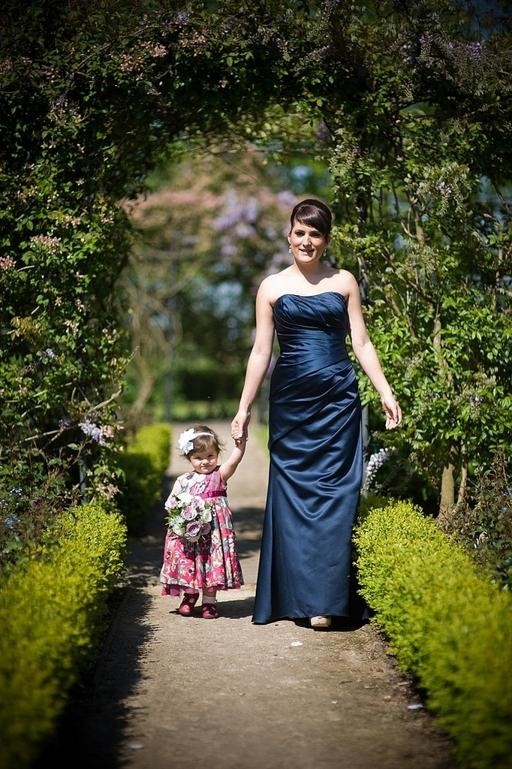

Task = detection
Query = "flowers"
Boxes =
[165,493,214,545]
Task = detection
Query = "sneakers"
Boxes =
[178,593,218,619]
[311,615,331,627]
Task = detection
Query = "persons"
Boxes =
[227,199,405,627]
[154,424,245,619]
